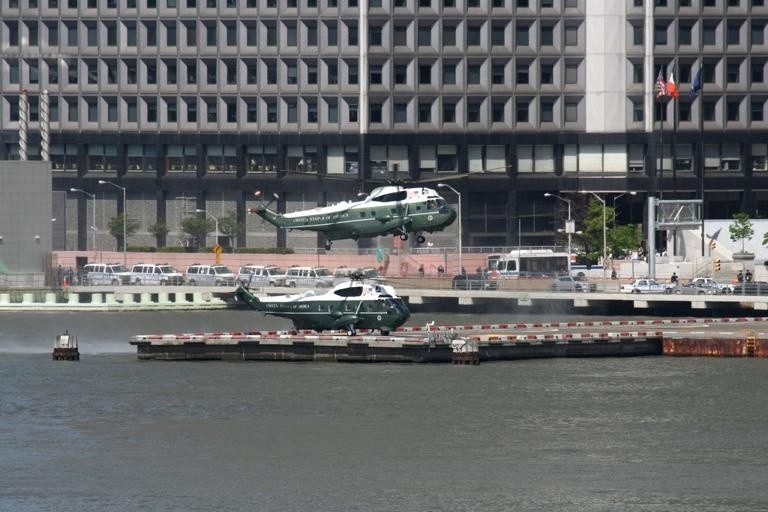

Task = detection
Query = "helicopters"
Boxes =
[244,164,515,250]
[209,266,413,336]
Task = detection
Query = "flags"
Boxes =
[687,55,703,100]
[665,54,681,99]
[654,63,666,100]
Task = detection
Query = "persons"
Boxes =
[476,265,481,274]
[55,264,63,286]
[438,264,444,274]
[75,267,85,284]
[65,266,75,284]
[744,269,752,283]
[671,272,678,283]
[492,267,497,277]
[737,269,743,283]
[418,264,425,277]
[483,267,489,276]
[461,266,466,274]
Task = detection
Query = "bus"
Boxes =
[485,247,574,281]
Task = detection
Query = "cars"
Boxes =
[617,277,675,294]
[549,275,597,294]
[690,277,738,295]
[731,281,768,296]
[670,282,722,297]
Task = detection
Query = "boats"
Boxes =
[50,328,81,362]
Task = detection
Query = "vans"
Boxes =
[331,267,388,288]
[86,263,131,286]
[184,264,238,286]
[284,267,335,288]
[451,273,498,291]
[129,263,185,286]
[238,267,285,286]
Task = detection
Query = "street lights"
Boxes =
[194,208,219,246]
[543,193,573,276]
[69,187,97,251]
[437,183,462,275]
[97,180,128,263]
[581,189,638,271]
